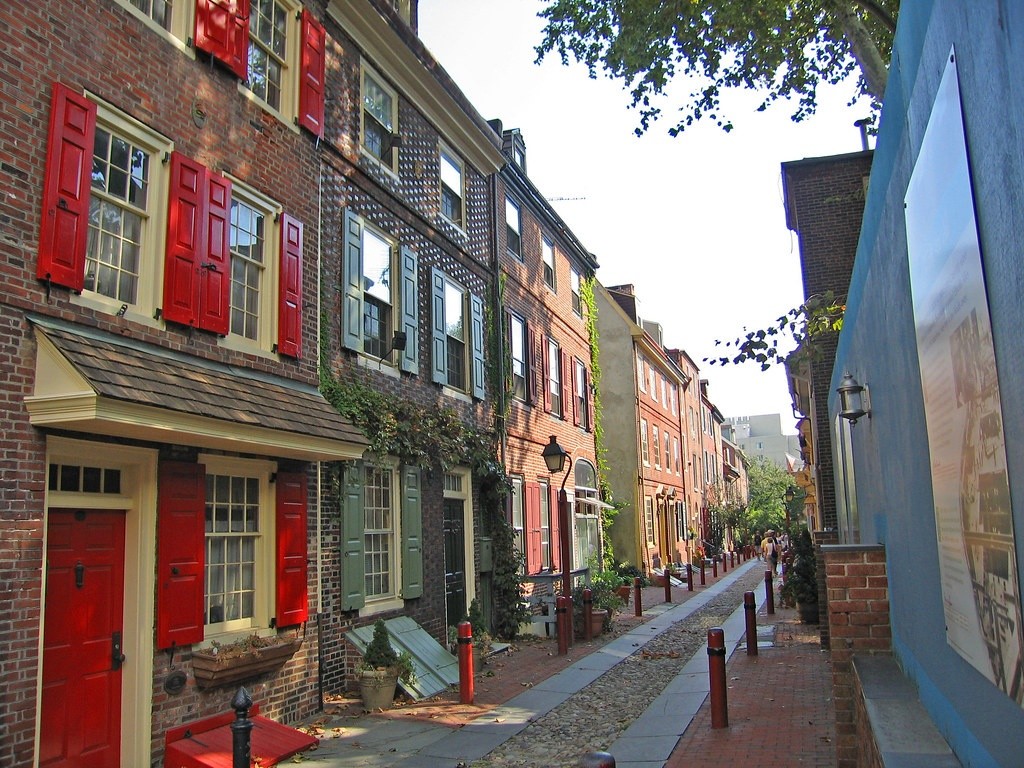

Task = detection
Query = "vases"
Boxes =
[656,576,665,587]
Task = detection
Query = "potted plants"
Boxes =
[789,529,819,625]
[613,576,631,607]
[574,570,606,636]
[458,596,487,671]
[669,564,681,579]
[599,570,622,630]
[353,617,417,712]
[192,630,305,691]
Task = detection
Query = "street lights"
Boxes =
[540,433,574,645]
[782,486,795,549]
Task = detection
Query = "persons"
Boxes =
[753,529,788,576]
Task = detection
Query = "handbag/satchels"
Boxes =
[771,544,778,559]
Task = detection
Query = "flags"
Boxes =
[785,454,795,471]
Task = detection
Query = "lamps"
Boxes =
[801,451,810,465]
[836,369,872,428]
[378,331,406,371]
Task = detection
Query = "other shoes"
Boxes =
[772,573,776,578]
